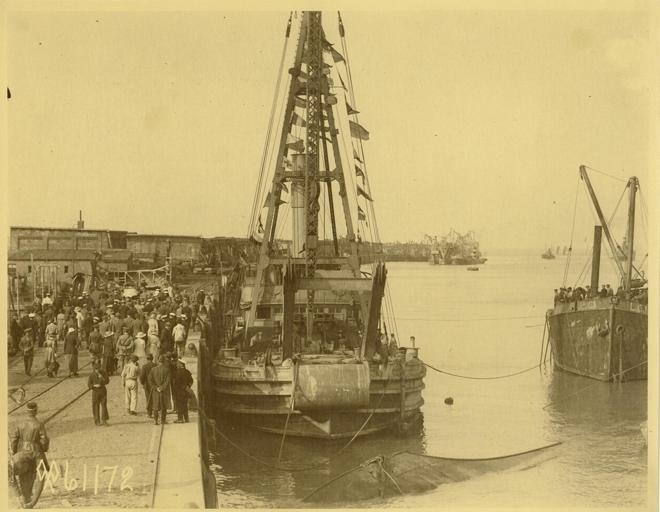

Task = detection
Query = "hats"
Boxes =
[93,317,99,321]
[123,327,129,332]
[69,328,75,333]
[24,328,32,332]
[46,293,51,296]
[92,360,101,369]
[151,313,187,320]
[104,332,113,337]
[106,305,114,309]
[74,307,81,312]
[26,402,39,410]
[135,332,146,338]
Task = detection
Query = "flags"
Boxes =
[284,132,305,153]
[249,232,263,247]
[330,45,346,65]
[349,120,370,141]
[291,111,306,127]
[281,155,294,171]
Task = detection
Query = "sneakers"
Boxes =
[97,420,108,426]
[128,410,189,425]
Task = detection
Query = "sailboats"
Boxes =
[191,8,425,446]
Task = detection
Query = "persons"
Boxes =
[10,278,206,430]
[552,283,614,307]
[9,401,50,510]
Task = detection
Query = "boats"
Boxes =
[429,230,637,264]
[541,162,648,387]
